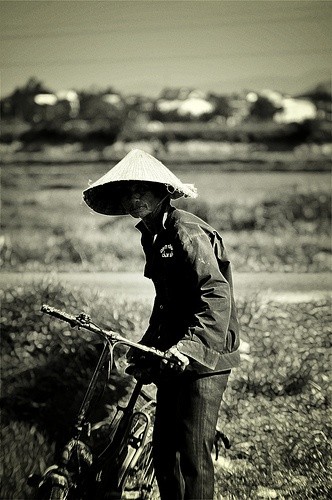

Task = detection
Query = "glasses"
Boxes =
[119,184,153,202]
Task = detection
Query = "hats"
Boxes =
[83,149,198,215]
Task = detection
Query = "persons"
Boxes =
[82,148,241,500]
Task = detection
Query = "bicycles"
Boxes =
[28,305,231,500]
